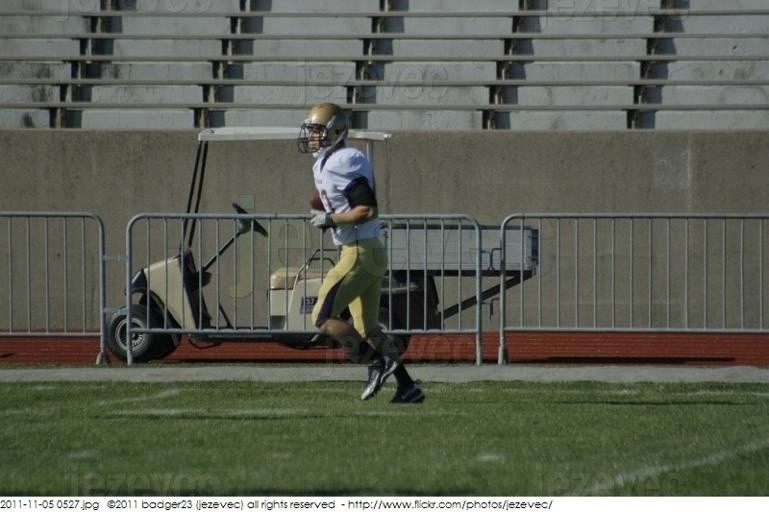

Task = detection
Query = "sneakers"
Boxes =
[360,351,426,405]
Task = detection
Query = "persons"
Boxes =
[295,101,427,404]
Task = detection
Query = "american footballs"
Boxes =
[309,191,325,210]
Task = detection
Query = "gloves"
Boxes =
[309,209,332,229]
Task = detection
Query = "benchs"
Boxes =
[2,1,767,133]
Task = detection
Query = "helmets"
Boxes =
[297,102,349,155]
